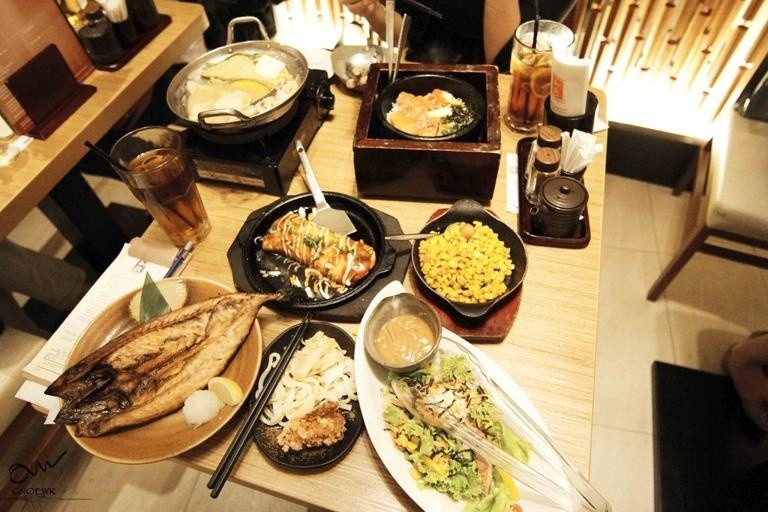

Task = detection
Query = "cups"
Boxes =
[364,296,442,373]
[560,149,589,180]
[525,174,586,238]
[544,89,600,140]
[107,129,209,253]
[502,19,576,133]
[74,0,159,61]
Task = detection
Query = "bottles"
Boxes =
[523,126,561,207]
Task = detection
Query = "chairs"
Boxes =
[647,52,768,303]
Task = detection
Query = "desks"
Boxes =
[1,0,211,339]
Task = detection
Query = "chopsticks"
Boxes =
[201,310,316,500]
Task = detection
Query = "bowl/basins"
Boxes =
[333,43,398,93]
[408,200,528,321]
[374,73,487,142]
[244,189,399,310]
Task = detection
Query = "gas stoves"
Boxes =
[131,62,340,195]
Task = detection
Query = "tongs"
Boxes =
[386,333,614,512]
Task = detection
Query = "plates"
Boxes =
[353,278,597,512]
[248,321,366,468]
[62,276,261,465]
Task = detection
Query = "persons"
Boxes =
[341,0,561,74]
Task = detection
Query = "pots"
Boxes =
[162,12,313,149]
